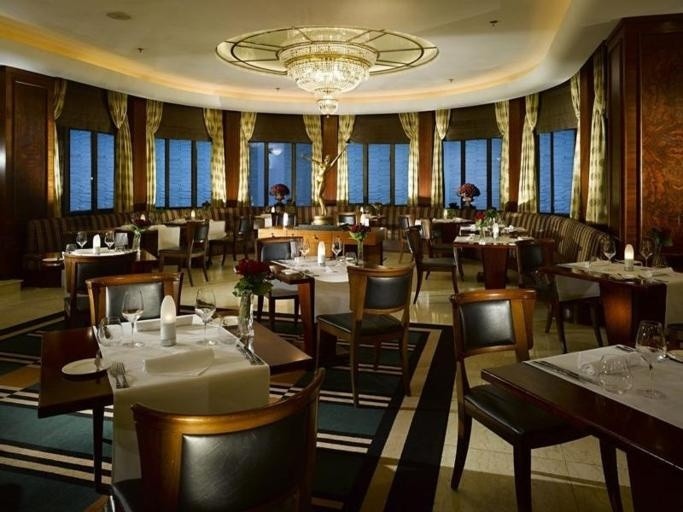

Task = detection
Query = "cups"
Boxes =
[597,354,632,394]
[346,252,356,264]
[98,317,122,346]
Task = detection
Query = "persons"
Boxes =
[300,145,346,216]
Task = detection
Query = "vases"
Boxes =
[461,194,472,209]
[275,194,284,206]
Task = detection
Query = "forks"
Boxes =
[109,362,129,389]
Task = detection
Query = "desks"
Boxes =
[38,317,312,490]
[482,334,681,512]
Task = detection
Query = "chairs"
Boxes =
[24,208,682,348]
[86,273,179,326]
[316,265,413,408]
[106,367,325,512]
[448,288,623,512]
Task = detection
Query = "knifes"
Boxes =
[94,349,102,382]
[235,344,264,366]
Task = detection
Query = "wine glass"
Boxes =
[121,289,146,349]
[298,239,310,267]
[604,241,617,271]
[195,288,217,345]
[635,319,667,399]
[638,240,653,271]
[75,231,87,253]
[104,231,115,252]
[331,238,343,267]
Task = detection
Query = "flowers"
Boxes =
[457,184,479,198]
[270,184,289,194]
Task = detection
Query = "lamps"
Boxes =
[278,42,377,119]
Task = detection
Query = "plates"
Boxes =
[62,359,111,376]
[668,349,683,363]
